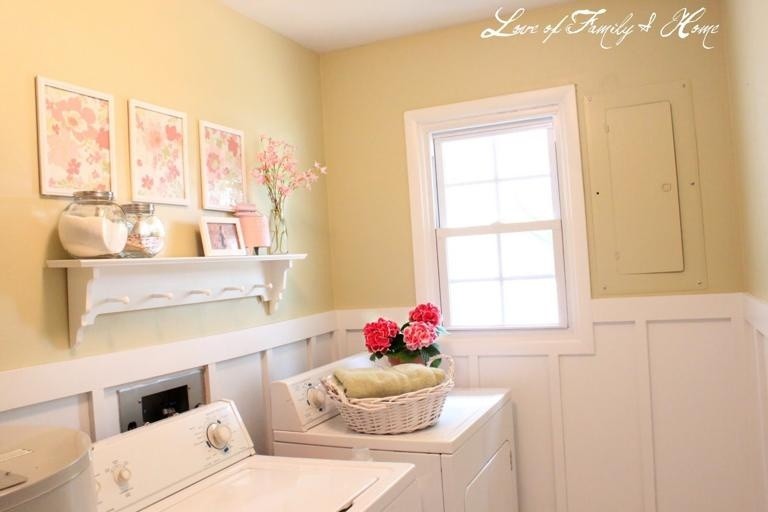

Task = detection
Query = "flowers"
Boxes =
[253,134,330,252]
[360,296,449,368]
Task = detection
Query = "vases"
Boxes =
[388,356,422,368]
[267,207,292,254]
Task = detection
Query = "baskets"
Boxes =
[318,353,456,437]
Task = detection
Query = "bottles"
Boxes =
[56,188,129,258]
[117,202,167,258]
[232,204,272,250]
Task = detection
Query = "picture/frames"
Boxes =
[197,118,250,214]
[35,76,118,202]
[127,97,193,209]
[196,212,247,257]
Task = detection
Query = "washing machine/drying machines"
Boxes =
[269,348,519,512]
[93,396,426,512]
